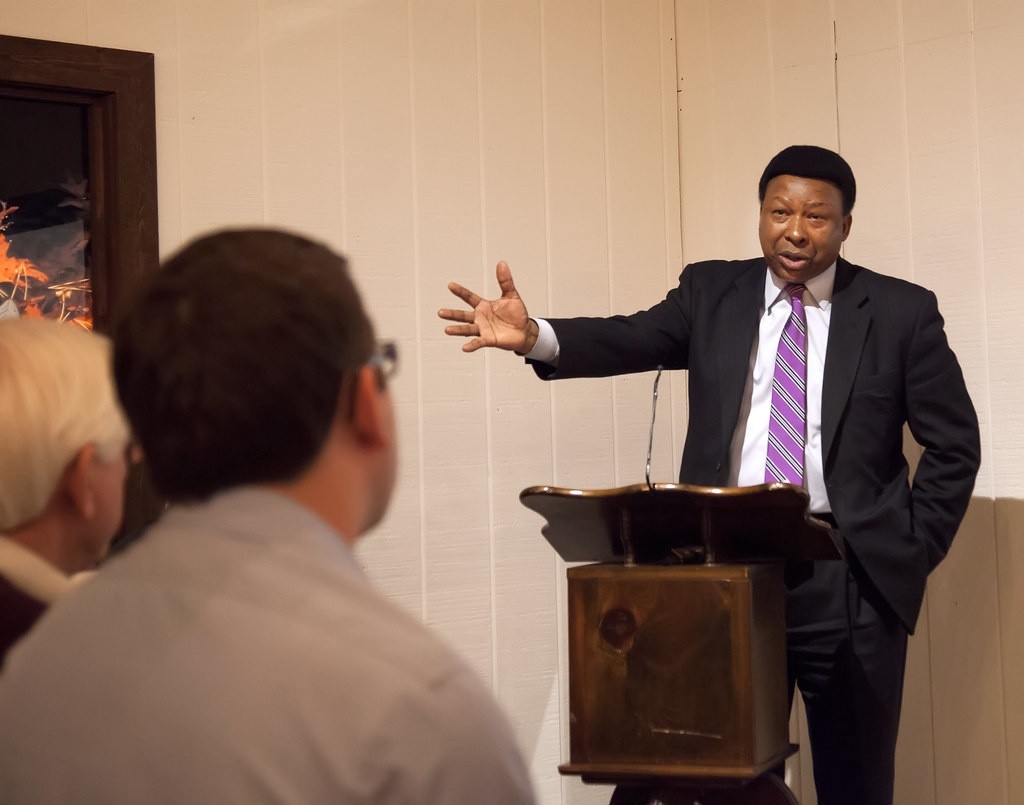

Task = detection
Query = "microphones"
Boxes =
[645,365,663,491]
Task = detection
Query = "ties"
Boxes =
[764,284,808,490]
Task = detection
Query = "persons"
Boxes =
[0,317,130,672]
[438,145,981,805]
[0,229,540,805]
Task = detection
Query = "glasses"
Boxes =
[124,437,147,468]
[346,342,400,423]
